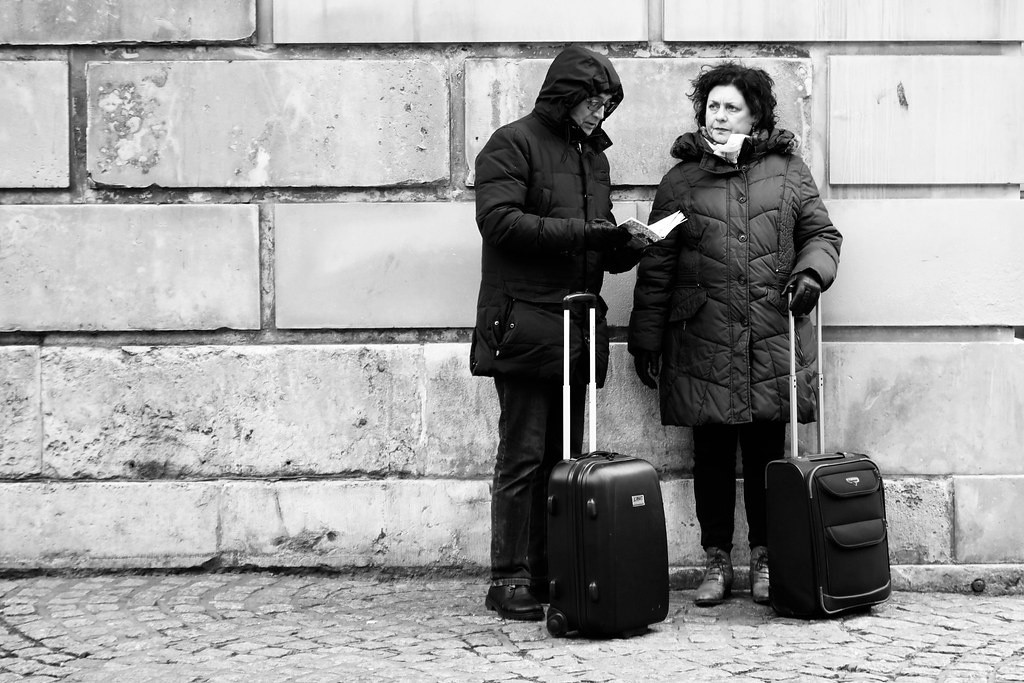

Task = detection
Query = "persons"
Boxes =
[627,63,843,607]
[469,46,647,621]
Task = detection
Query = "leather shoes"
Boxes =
[749,546,769,603]
[485,584,545,621]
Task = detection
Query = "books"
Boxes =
[616,210,688,249]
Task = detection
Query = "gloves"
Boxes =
[781,271,821,317]
[585,218,633,250]
[634,350,661,389]
[609,245,649,274]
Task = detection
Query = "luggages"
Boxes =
[546,289,670,641]
[764,283,894,620]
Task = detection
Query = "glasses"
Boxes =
[586,98,617,113]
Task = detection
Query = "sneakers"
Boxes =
[693,546,734,607]
